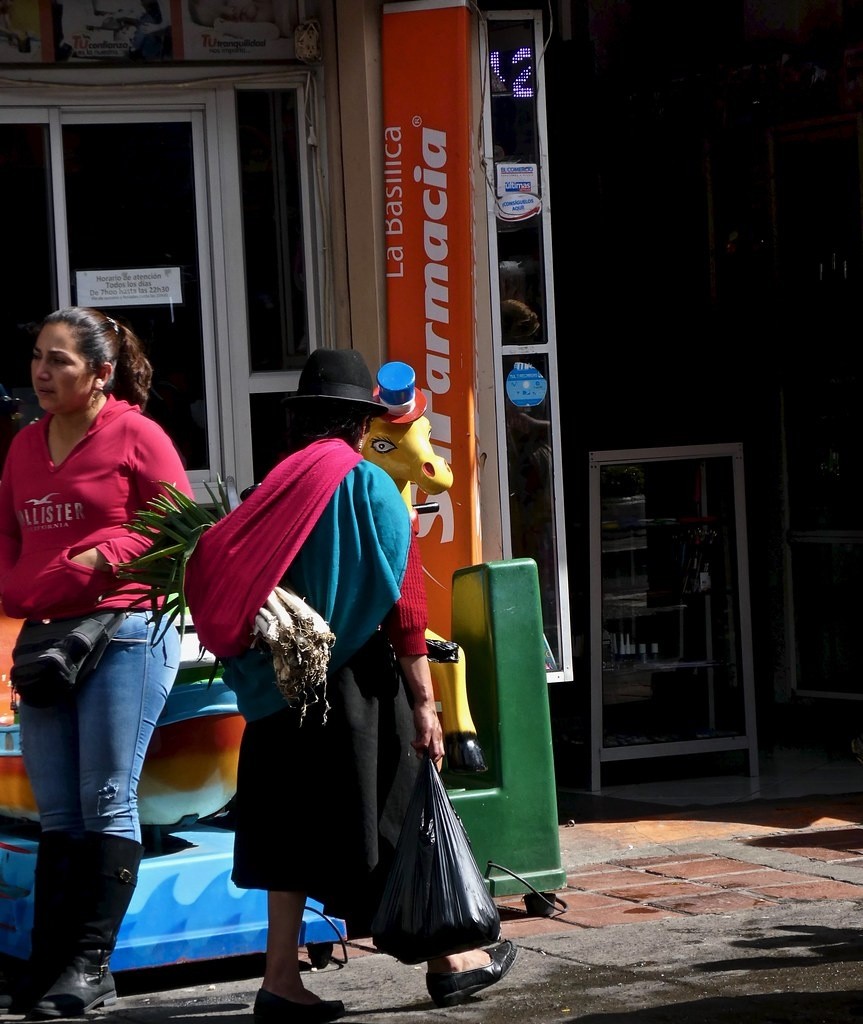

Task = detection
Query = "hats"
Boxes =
[279,347,390,419]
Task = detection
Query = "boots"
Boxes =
[7,833,84,1016]
[24,831,145,1020]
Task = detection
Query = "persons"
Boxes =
[0,306,197,1017]
[189,0,292,40]
[230,346,518,1024]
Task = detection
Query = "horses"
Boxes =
[357,414,489,775]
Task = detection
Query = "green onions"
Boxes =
[104,473,337,695]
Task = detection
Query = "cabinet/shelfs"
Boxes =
[482,5,573,684]
[573,443,760,796]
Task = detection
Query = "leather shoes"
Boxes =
[425,939,518,1009]
[252,985,345,1024]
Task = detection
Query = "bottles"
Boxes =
[601,629,659,672]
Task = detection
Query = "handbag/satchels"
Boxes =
[374,747,501,964]
[9,606,138,713]
